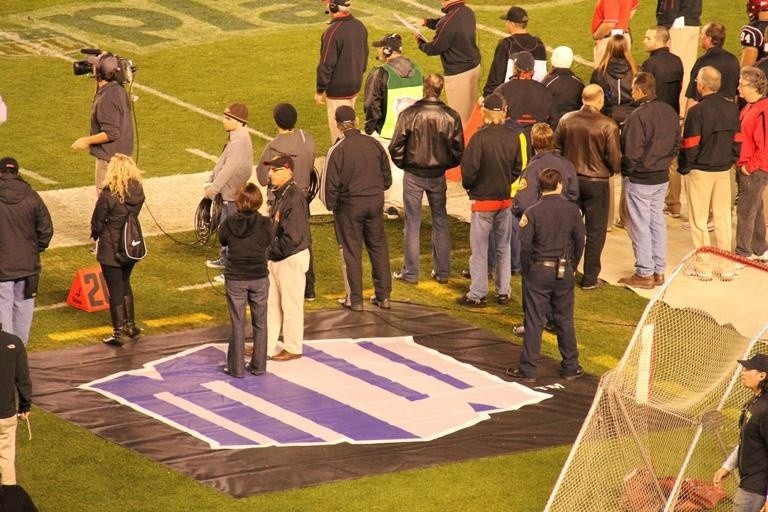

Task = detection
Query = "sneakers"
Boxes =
[513,326,525,336]
[581,275,664,288]
[204,259,225,269]
[215,275,226,282]
[681,222,715,231]
[456,269,510,307]
[684,250,768,281]
[385,207,398,219]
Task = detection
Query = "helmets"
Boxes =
[746,0,768,23]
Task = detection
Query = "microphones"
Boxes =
[326,11,329,13]
[376,56,379,60]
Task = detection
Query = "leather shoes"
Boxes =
[370,296,391,308]
[394,271,420,284]
[224,345,301,378]
[431,269,448,284]
[563,365,584,380]
[507,368,536,383]
[343,297,364,311]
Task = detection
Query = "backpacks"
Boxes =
[113,215,147,265]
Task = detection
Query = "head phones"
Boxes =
[95,52,113,81]
[329,0,346,13]
[383,33,401,57]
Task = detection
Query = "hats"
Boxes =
[510,51,535,71]
[551,47,574,69]
[264,155,294,170]
[0,157,18,175]
[224,103,249,123]
[273,103,297,129]
[481,93,506,111]
[372,34,402,51]
[500,7,528,23]
[336,105,356,123]
[738,355,767,372]
[323,0,350,6]
[88,53,118,73]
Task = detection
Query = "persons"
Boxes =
[0,323,32,484]
[200,3,768,384]
[0,475,40,512]
[88,153,146,347]
[70,53,133,193]
[0,157,54,347]
[713,353,768,512]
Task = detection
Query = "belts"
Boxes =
[532,261,558,267]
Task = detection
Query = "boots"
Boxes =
[124,295,140,338]
[102,304,124,346]
